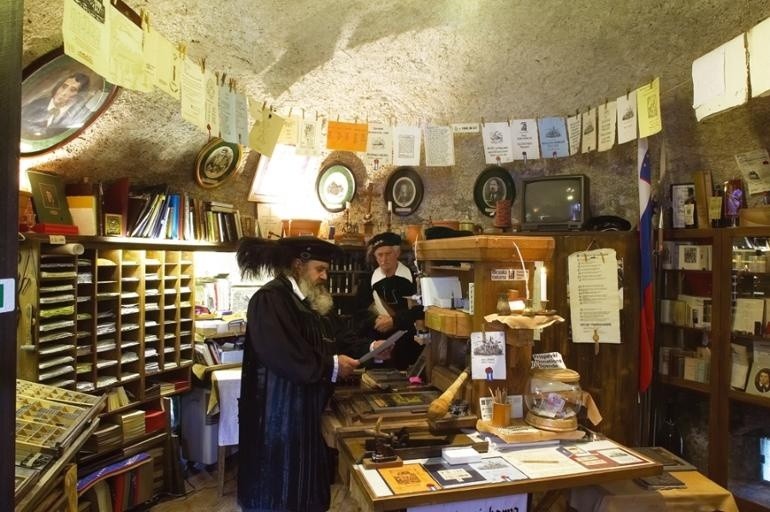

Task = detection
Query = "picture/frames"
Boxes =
[632,446,699,471]
[383,165,424,219]
[102,210,125,237]
[670,184,698,227]
[227,284,261,312]
[633,470,687,491]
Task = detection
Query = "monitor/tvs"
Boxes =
[521,173,590,231]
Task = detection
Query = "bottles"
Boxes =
[762,191,770,207]
[684,188,697,230]
[712,184,726,227]
[496,290,550,315]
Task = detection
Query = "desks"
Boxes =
[310,388,665,510]
[15,415,101,510]
[569,445,739,511]
[213,367,247,496]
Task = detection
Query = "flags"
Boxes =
[638,148,655,393]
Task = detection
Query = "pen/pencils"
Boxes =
[488,386,507,404]
[523,459,559,463]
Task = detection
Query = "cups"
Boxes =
[490,401,512,427]
[459,222,474,234]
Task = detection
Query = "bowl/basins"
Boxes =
[525,366,583,419]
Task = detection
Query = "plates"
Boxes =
[385,167,423,216]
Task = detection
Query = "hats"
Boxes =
[369,231,403,253]
[280,234,342,266]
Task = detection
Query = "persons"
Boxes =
[206,149,229,175]
[20,74,89,141]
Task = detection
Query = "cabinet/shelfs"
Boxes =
[647,225,769,510]
[325,248,418,349]
[16,231,197,406]
[415,233,558,421]
[69,396,187,511]
[561,229,648,448]
